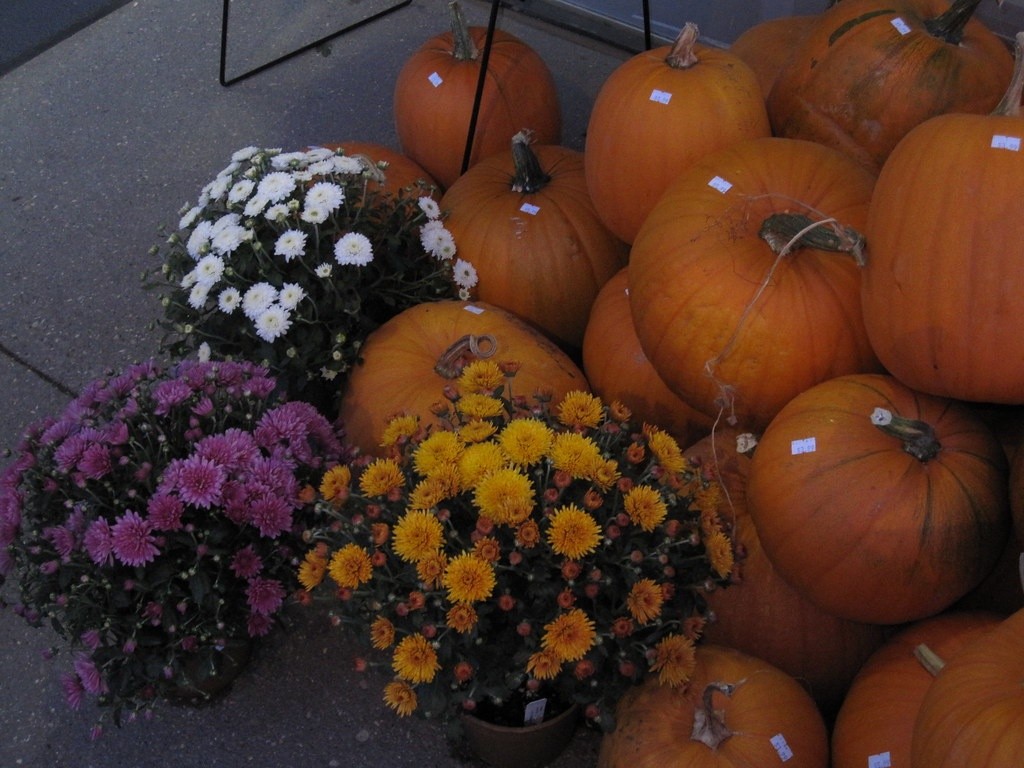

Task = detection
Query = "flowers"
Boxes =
[296,361,749,724]
[138,143,480,409]
[0,360,343,742]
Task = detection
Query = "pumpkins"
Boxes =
[299,0,1024,768]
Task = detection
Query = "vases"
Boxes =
[167,639,247,706]
[455,695,584,768]
